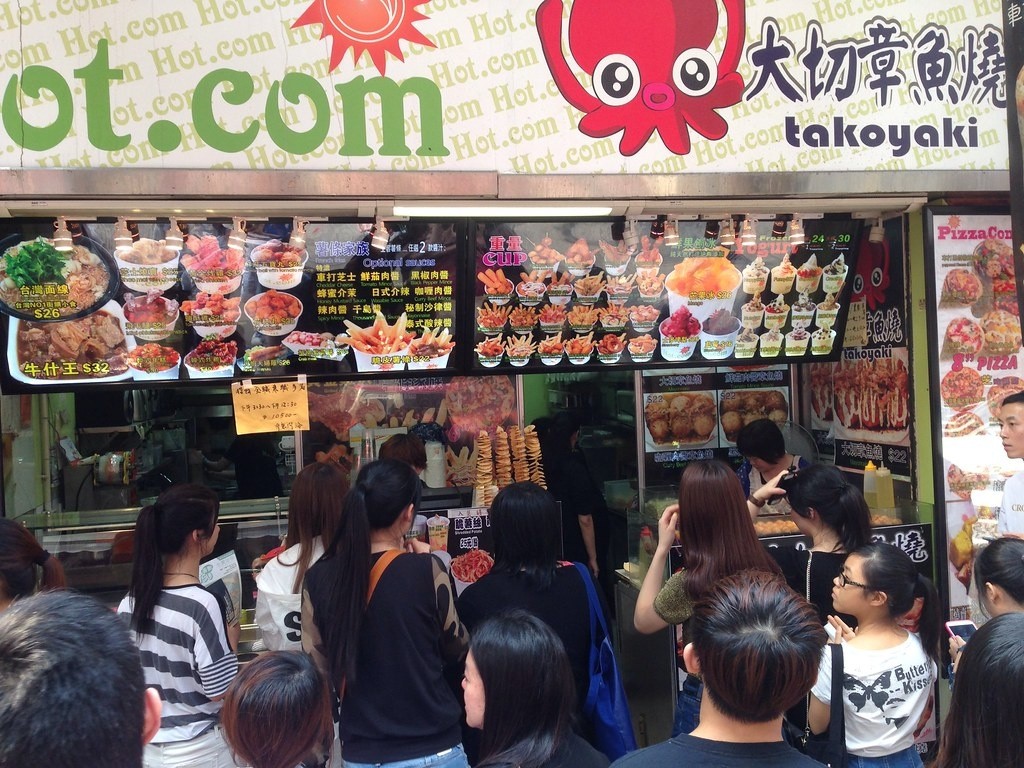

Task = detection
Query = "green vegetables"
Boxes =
[4,237,75,290]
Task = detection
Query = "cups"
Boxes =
[426,516,450,552]
[358,427,446,488]
[350,326,412,372]
[407,338,452,369]
[403,515,427,546]
[451,555,494,598]
[477,246,848,367]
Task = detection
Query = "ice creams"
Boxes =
[735,252,848,341]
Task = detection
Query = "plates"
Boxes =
[938,236,1023,502]
[281,331,349,362]
[644,390,717,450]
[718,389,789,447]
[7,299,138,386]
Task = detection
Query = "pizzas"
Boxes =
[946,238,1024,436]
[444,376,515,433]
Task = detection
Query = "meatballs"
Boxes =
[647,391,716,440]
[750,519,799,534]
[721,391,789,434]
[872,515,901,525]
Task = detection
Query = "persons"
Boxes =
[301,460,470,768]
[0,591,161,768]
[199,429,283,497]
[745,465,872,730]
[114,484,240,768]
[378,435,426,474]
[734,419,811,517]
[255,462,349,768]
[610,570,830,768]
[808,544,941,768]
[933,391,1024,768]
[0,517,67,611]
[635,460,785,738]
[456,480,612,768]
[222,649,333,768]
[461,610,610,768]
[544,411,611,588]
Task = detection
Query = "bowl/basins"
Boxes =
[115,243,308,381]
[0,231,120,322]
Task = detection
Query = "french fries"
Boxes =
[335,310,456,359]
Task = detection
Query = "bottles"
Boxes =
[875,461,896,508]
[863,460,877,509]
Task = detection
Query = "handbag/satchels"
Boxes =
[574,562,636,759]
[786,644,847,768]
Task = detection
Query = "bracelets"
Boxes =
[203,457,207,462]
[748,493,766,507]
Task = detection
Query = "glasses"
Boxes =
[837,568,878,594]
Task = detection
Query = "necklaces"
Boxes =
[163,572,200,582]
